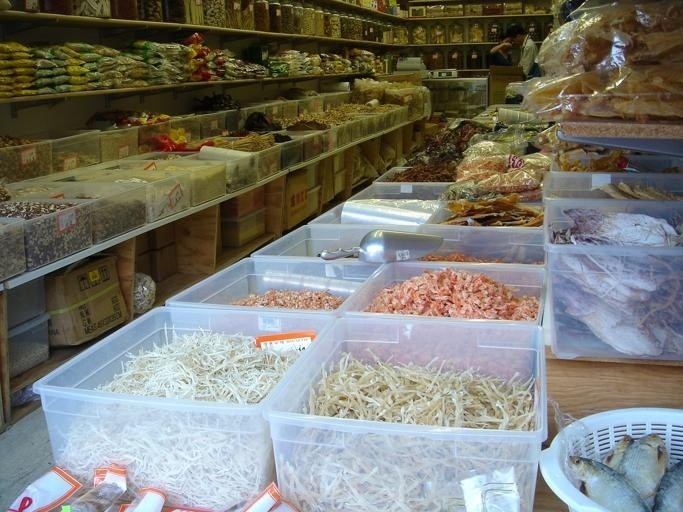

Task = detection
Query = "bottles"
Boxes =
[0,0,558,45]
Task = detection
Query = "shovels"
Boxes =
[321,229,443,263]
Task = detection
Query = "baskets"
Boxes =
[538,407,682,512]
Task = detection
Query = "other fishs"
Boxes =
[550,207,683,358]
[567,432,683,512]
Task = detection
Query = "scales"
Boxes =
[432,69,457,78]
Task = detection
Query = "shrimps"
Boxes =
[216,287,348,312]
[360,266,541,386]
[416,252,544,265]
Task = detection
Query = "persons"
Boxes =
[501,24,541,83]
